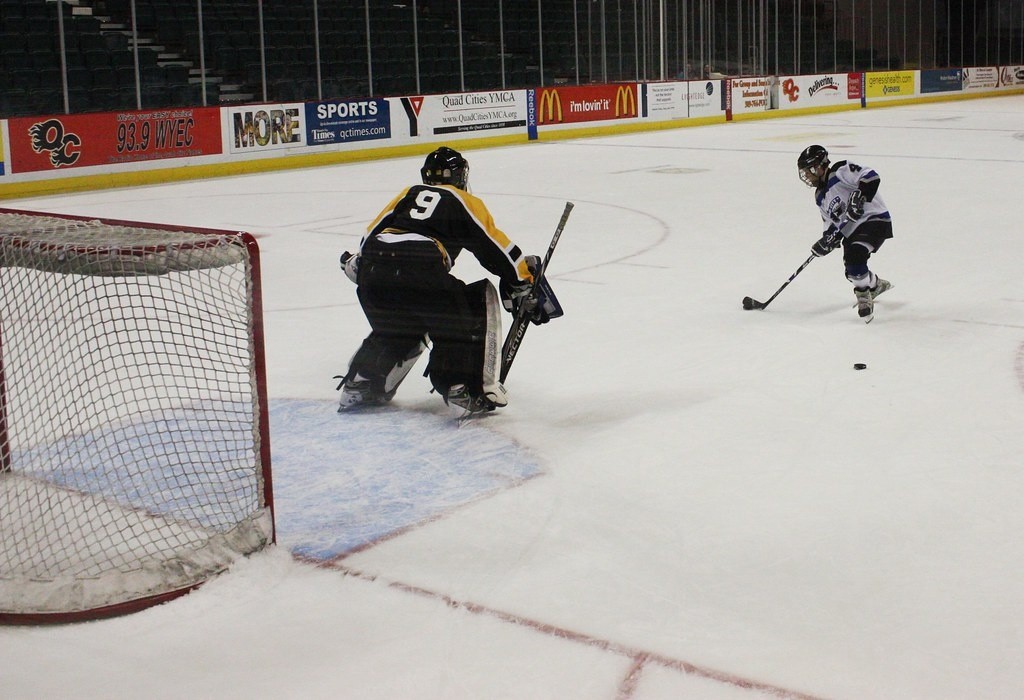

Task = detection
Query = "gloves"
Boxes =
[847,189,864,221]
[811,235,838,258]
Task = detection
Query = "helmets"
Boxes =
[798,146,830,188]
[421,147,469,192]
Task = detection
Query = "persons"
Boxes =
[679,62,717,80]
[796,144,893,323]
[336,145,550,411]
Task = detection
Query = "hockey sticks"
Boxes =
[742,195,867,312]
[499,200,576,385]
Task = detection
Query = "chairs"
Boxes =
[0,0,1023,118]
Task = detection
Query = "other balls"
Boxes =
[742,305,754,311]
[854,363,867,370]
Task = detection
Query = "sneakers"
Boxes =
[444,381,487,414]
[853,287,874,324]
[340,380,389,407]
[869,274,894,302]
